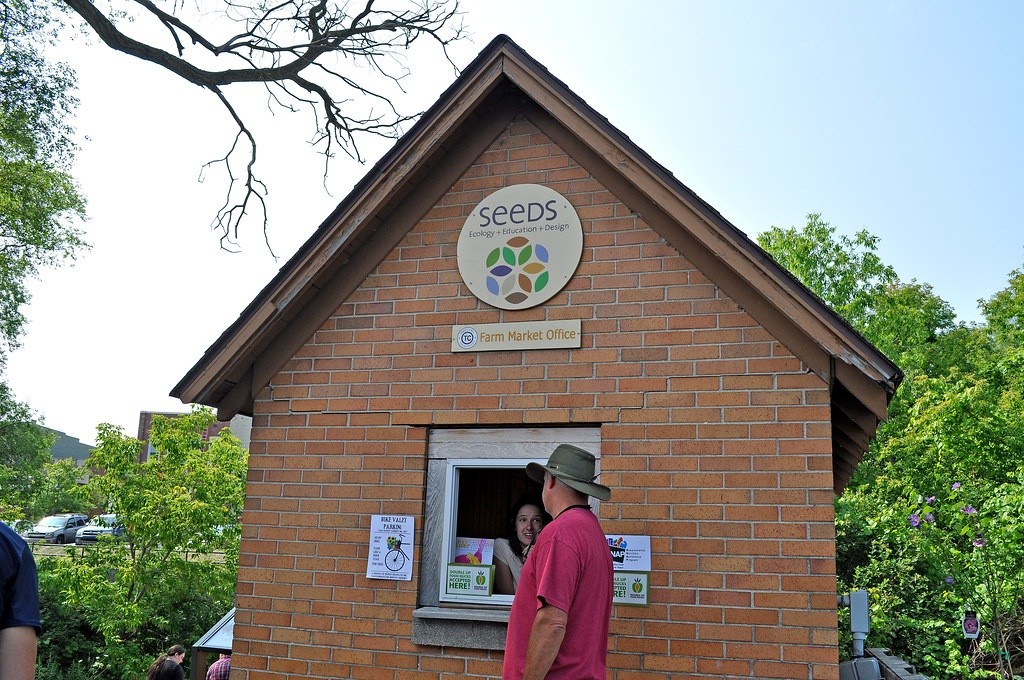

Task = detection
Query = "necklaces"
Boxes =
[555,505,591,519]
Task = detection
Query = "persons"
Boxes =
[206,653,231,680]
[494,496,553,595]
[502,444,614,680]
[0,522,42,680]
[146,645,186,680]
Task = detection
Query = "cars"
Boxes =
[20,512,88,544]
[75,513,130,546]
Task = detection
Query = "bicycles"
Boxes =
[385,534,411,572]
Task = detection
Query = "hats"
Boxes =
[526,444,612,502]
